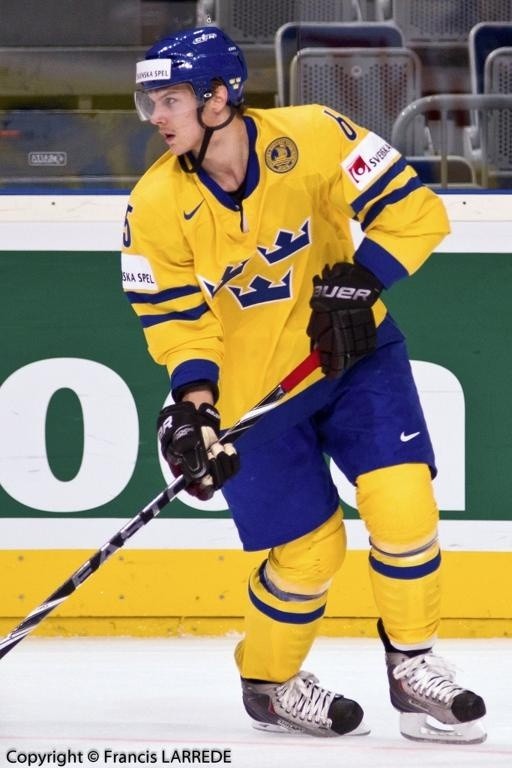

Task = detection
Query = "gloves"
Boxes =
[304,259,385,382]
[156,402,240,501]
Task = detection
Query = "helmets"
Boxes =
[131,26,249,123]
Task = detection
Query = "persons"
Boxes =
[119,26,488,740]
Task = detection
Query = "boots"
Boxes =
[376,616,487,726]
[240,675,364,738]
